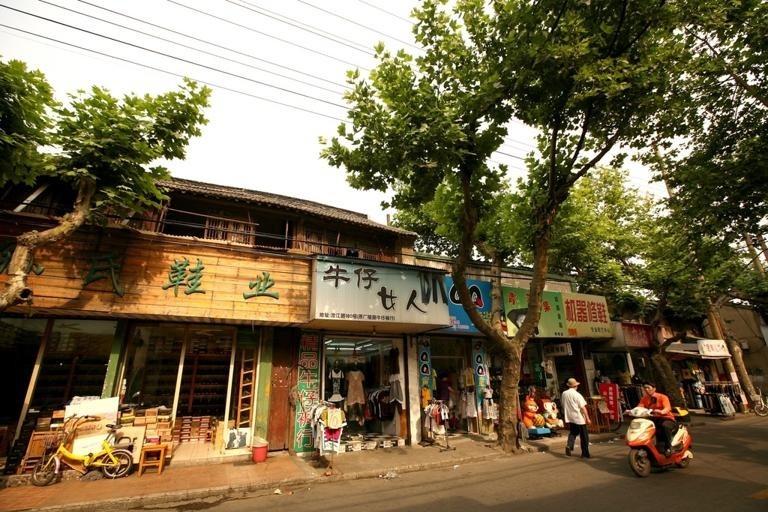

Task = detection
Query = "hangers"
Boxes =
[333,366,341,371]
[427,399,446,407]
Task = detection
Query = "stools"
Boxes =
[137,445,169,477]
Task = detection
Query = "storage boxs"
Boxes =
[35,401,215,461]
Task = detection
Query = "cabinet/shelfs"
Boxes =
[190,351,235,414]
[33,351,78,408]
[137,353,196,415]
[62,354,107,407]
[584,395,610,433]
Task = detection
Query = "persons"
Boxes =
[560,377,592,459]
[440,377,460,401]
[624,380,678,457]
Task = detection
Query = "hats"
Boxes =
[566,378,581,387]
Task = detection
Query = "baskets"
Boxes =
[750,395,760,401]
[43,435,62,448]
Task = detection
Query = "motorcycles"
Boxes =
[623,403,696,480]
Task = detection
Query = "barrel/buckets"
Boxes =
[252,444,268,463]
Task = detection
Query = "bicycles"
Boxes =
[611,408,622,432]
[33,413,138,486]
[753,386,768,417]
